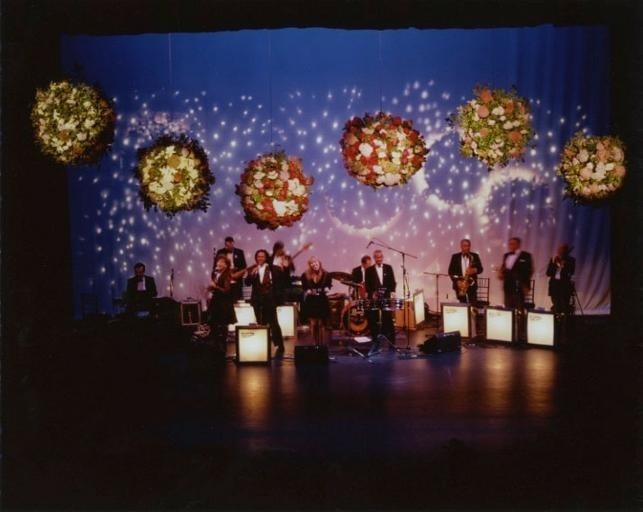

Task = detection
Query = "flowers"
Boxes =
[561,133,624,199]
[339,112,429,189]
[135,136,212,212]
[453,90,534,169]
[237,153,311,230]
[35,81,116,168]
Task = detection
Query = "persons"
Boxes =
[244,249,283,352]
[212,236,246,303]
[351,255,372,285]
[204,257,233,353]
[263,240,295,303]
[495,237,534,309]
[124,263,156,297]
[299,256,336,361]
[545,241,577,316]
[364,248,396,350]
[447,239,483,303]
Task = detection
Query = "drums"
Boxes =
[342,301,370,335]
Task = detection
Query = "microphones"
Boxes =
[366,240,374,249]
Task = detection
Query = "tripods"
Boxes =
[330,241,422,364]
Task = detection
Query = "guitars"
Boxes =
[230,265,255,284]
[278,242,311,271]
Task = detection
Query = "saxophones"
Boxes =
[458,251,474,296]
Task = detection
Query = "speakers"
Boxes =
[419,331,461,354]
[294,345,328,369]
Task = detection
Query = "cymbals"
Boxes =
[328,272,353,281]
[340,280,363,288]
